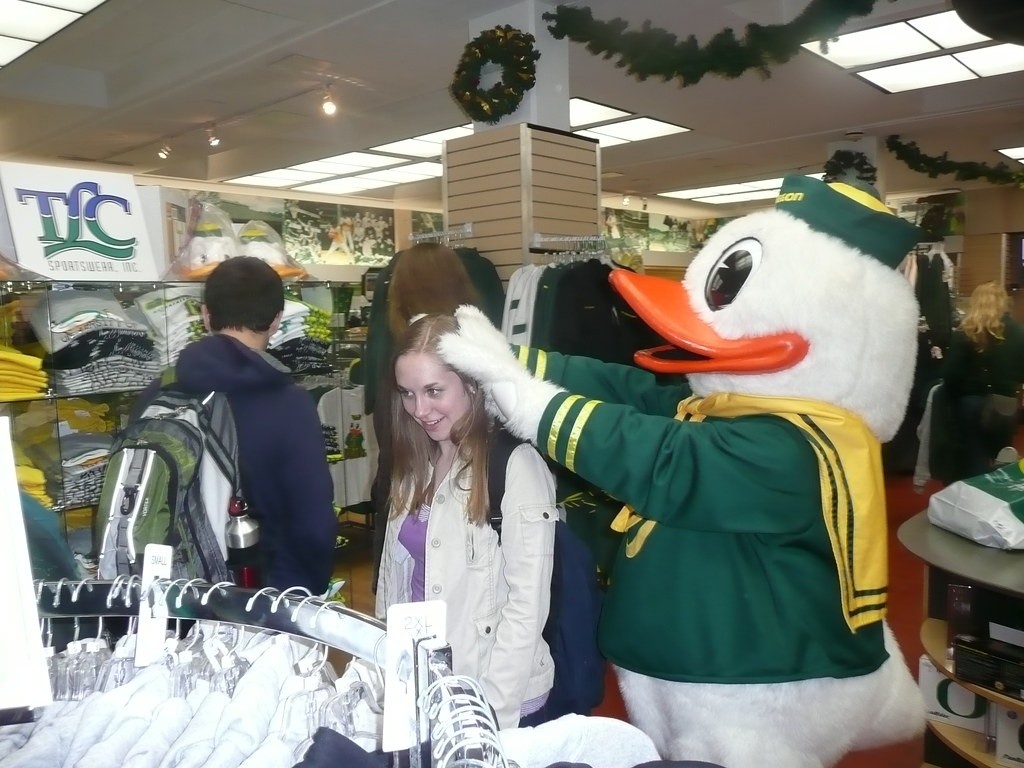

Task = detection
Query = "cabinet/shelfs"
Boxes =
[897,511,1024,768]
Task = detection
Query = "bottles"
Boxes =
[225,497,265,588]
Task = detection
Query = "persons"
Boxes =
[388,241,477,337]
[130,258,335,598]
[945,282,1024,395]
[371,314,559,727]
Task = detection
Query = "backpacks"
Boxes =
[93,366,242,584]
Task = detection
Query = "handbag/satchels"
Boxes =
[925,458,1024,549]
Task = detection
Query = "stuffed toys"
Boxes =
[177,222,240,279]
[237,226,306,277]
[410,174,928,768]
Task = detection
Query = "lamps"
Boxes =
[158,141,175,159]
[641,197,649,211]
[203,124,222,147]
[318,86,337,114]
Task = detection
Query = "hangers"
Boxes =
[33,575,506,768]
